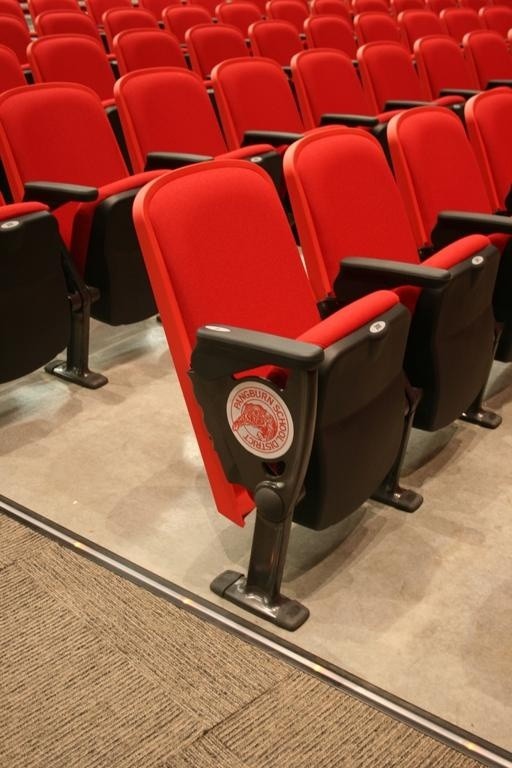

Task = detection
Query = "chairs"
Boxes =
[0,1,511,634]
